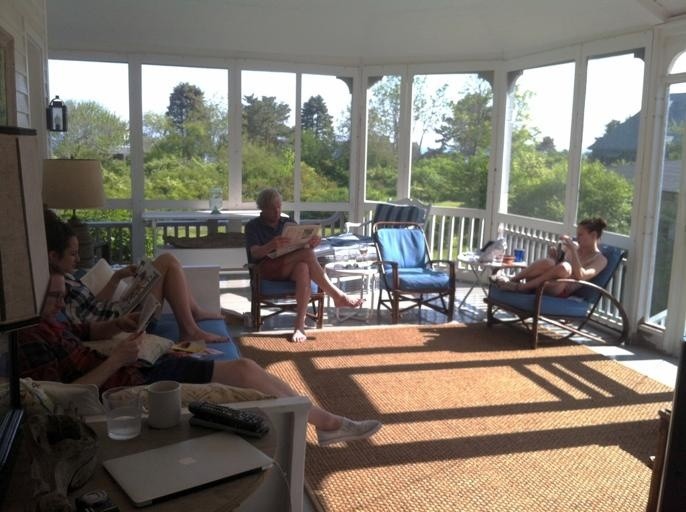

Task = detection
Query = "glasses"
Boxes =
[48,292,63,303]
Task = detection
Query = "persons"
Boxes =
[6,262,382,449]
[41,204,230,345]
[499,217,609,297]
[243,188,366,343]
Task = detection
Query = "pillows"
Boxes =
[18,378,102,415]
[109,382,278,408]
[82,331,175,366]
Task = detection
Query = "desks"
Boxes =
[0,407,278,512]
[141,210,290,269]
[324,261,380,323]
[456,252,527,311]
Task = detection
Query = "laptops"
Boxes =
[101,430,275,506]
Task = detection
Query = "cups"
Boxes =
[102,380,182,440]
[514,249,524,261]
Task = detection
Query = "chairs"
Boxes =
[300,208,372,249]
[483,239,629,349]
[247,216,326,331]
[372,221,458,323]
[343,198,433,246]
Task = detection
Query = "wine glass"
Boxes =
[359,241,368,267]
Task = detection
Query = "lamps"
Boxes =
[47,95,67,132]
[43,154,107,262]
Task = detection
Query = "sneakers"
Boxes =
[489,275,520,291]
[316,416,381,446]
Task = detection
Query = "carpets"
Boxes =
[233,319,675,512]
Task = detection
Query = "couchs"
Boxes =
[0,265,312,512]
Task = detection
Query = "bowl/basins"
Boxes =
[497,256,516,265]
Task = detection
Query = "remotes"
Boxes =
[187,400,265,430]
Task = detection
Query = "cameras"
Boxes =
[76,489,119,512]
